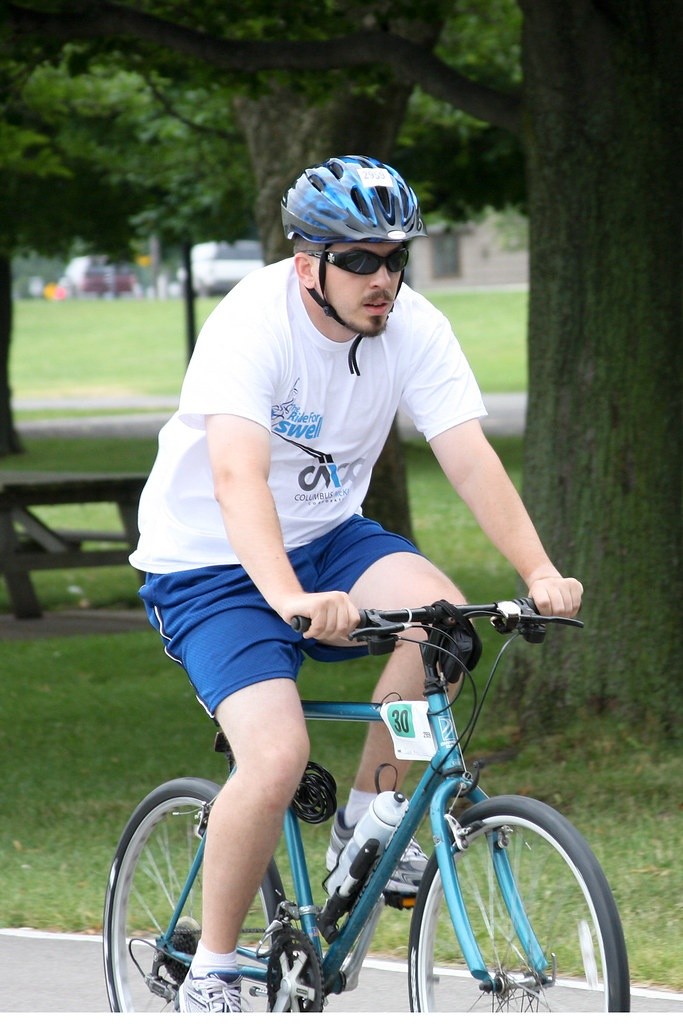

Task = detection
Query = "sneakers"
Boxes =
[327,811,431,911]
[178,967,243,1013]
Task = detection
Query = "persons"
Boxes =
[125,155,591,1013]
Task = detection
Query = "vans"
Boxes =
[177,236,266,296]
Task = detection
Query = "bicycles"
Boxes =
[100,594,636,1012]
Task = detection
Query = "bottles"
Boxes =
[324,792,410,905]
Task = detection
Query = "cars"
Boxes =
[60,253,136,300]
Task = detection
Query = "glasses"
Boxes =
[299,249,410,276]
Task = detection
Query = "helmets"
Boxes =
[281,154,427,244]
